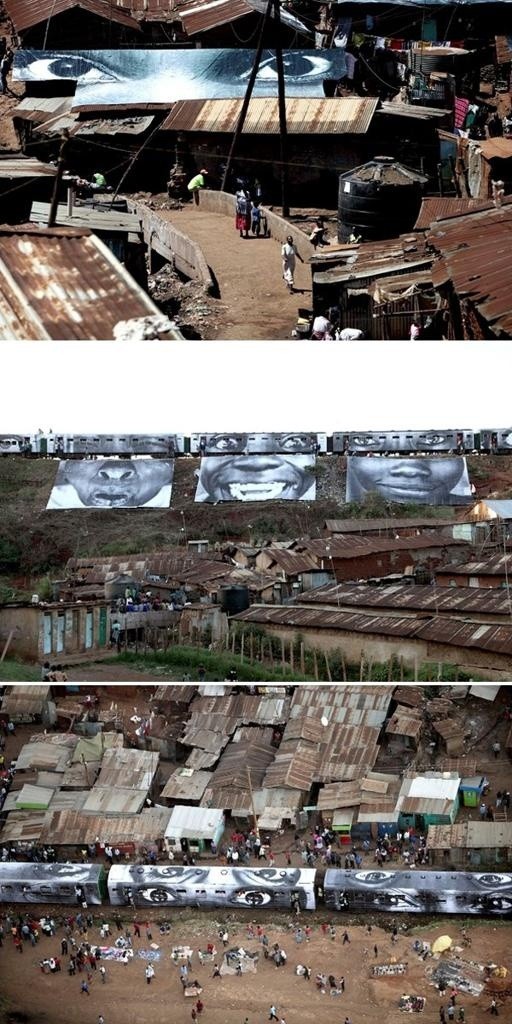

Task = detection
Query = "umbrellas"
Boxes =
[431,934,453,953]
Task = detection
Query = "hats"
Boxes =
[200,169,208,174]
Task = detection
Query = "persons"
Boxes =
[478,783,511,822]
[223,664,238,681]
[199,434,318,503]
[41,661,50,681]
[58,436,175,512]
[408,317,423,340]
[448,1004,456,1020]
[50,664,67,682]
[13,46,349,99]
[1,715,17,823]
[3,819,431,1024]
[450,988,458,1006]
[439,981,446,998]
[116,585,186,613]
[92,172,108,193]
[460,926,472,949]
[44,665,55,682]
[339,429,477,508]
[494,695,512,720]
[288,305,368,339]
[144,797,200,809]
[251,197,267,238]
[109,627,120,648]
[489,996,499,1016]
[456,1007,467,1024]
[493,740,501,758]
[439,1005,448,1024]
[182,671,193,682]
[235,179,252,239]
[273,731,281,747]
[186,168,211,193]
[308,218,330,252]
[197,663,207,682]
[281,234,305,295]
[30,591,99,607]
[420,542,493,570]
[79,690,100,711]
[111,619,121,632]
